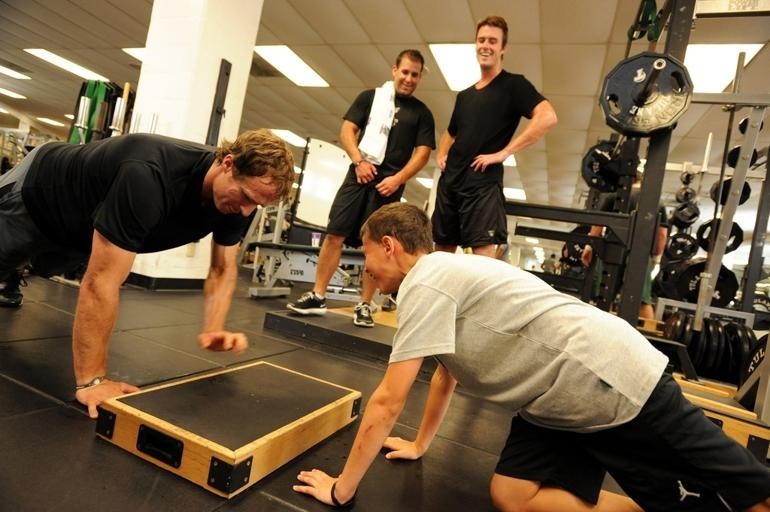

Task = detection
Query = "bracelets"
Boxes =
[330,477,358,508]
[353,159,367,168]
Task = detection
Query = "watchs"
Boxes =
[74,376,108,392]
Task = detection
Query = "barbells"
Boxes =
[581,51,694,193]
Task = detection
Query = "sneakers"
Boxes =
[0,264,24,308]
[354,302,374,327]
[286,292,327,315]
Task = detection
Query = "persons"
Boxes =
[292,201,769,512]
[543,250,561,276]
[581,170,671,320]
[0,128,293,420]
[286,49,437,327]
[431,14,558,261]
